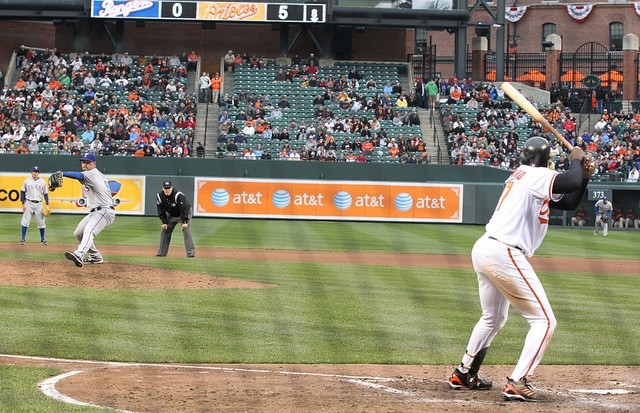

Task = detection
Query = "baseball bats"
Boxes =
[501,81,596,171]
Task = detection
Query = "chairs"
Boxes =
[0,53,195,157]
[439,80,639,181]
[219,59,428,164]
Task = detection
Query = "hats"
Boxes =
[79,151,96,161]
[603,195,608,199]
[31,165,39,172]
[163,180,172,188]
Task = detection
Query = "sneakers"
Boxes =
[64,249,83,266]
[41,239,47,245]
[449,368,493,390]
[21,239,26,244]
[83,256,103,264]
[502,376,548,401]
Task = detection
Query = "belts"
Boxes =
[26,198,43,203]
[90,205,115,212]
[488,235,526,252]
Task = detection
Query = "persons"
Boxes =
[300,79,308,88]
[407,90,416,107]
[333,82,346,91]
[333,76,342,85]
[324,88,332,100]
[341,74,347,82]
[392,82,402,94]
[397,89,409,107]
[349,78,360,90]
[439,79,572,172]
[18,166,52,245]
[324,107,333,117]
[240,49,248,59]
[383,82,393,95]
[211,74,215,78]
[59,152,117,267]
[614,208,623,228]
[306,61,319,75]
[612,210,615,227]
[307,52,319,66]
[396,95,407,108]
[235,54,243,65]
[329,93,339,105]
[274,68,285,81]
[207,72,209,77]
[291,64,299,77]
[0,45,198,158]
[347,65,365,80]
[292,54,302,65]
[573,108,640,184]
[326,75,334,90]
[365,79,377,91]
[300,64,309,75]
[317,77,325,87]
[224,50,235,72]
[381,129,428,167]
[570,205,587,227]
[250,54,261,70]
[593,84,606,115]
[593,196,613,237]
[375,106,420,127]
[339,89,375,113]
[634,219,640,228]
[318,116,380,163]
[419,77,427,108]
[308,76,317,88]
[216,93,324,163]
[211,71,223,103]
[198,71,211,103]
[384,95,395,107]
[415,76,421,109]
[154,179,198,259]
[284,70,292,82]
[196,141,206,158]
[448,135,585,403]
[619,208,635,229]
[375,92,384,106]
[434,76,440,101]
[425,78,438,111]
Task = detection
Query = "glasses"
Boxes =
[81,160,93,163]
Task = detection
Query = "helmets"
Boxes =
[522,135,551,166]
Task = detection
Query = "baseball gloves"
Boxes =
[42,205,51,217]
[48,170,63,191]
